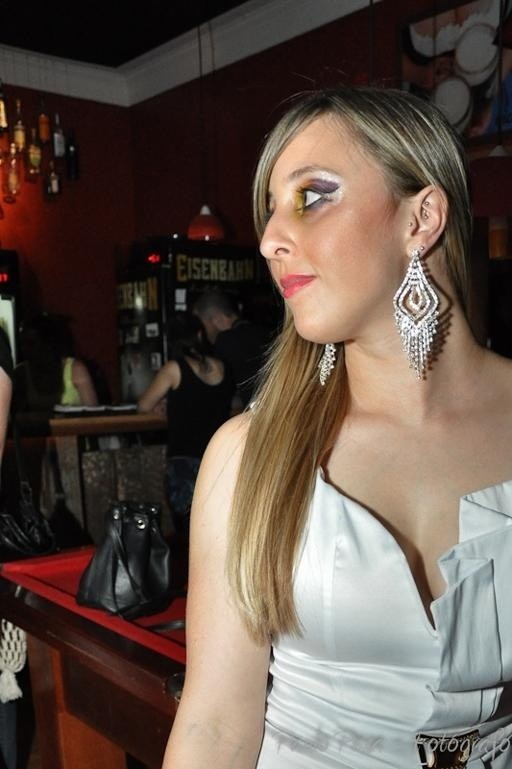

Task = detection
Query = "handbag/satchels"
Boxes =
[76,499,182,633]
[0,491,61,561]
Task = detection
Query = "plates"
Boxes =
[435,77,475,136]
[452,22,501,88]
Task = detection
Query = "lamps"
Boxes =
[468,0,511,219]
[188,0,224,242]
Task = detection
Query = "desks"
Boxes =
[0,543,188,769]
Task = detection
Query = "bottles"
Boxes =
[0,76,82,200]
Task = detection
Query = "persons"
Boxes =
[185,287,270,416]
[159,79,512,769]
[0,326,27,769]
[19,313,99,406]
[137,309,235,533]
